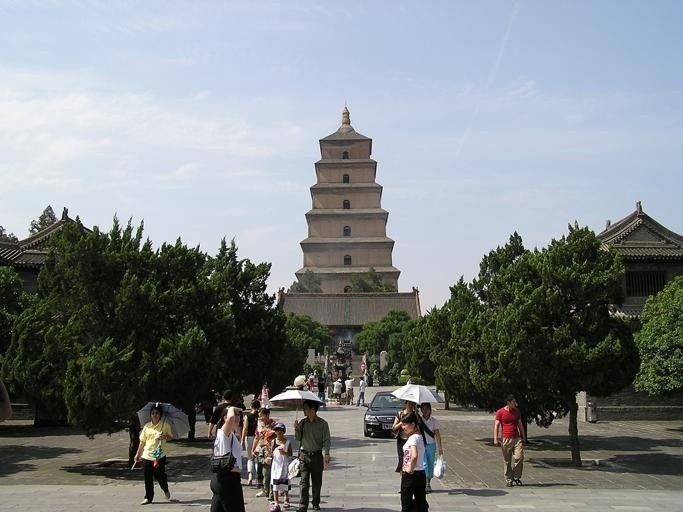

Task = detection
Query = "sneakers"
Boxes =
[140,499,152,505]
[163,489,170,499]
[248,479,289,512]
[426,487,431,494]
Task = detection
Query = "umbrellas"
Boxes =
[390,384,445,414]
[136,401,191,440]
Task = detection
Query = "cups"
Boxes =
[277,445,283,451]
[266,457,272,464]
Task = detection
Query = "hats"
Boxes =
[266,431,275,440]
[272,422,286,430]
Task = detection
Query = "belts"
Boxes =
[304,452,317,456]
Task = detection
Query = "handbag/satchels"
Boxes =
[395,428,401,439]
[288,455,302,478]
[210,452,237,473]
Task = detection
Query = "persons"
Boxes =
[494,397,526,487]
[420,403,443,492]
[207,370,368,512]
[134,405,173,505]
[0,379,13,419]
[392,400,420,493]
[400,413,429,512]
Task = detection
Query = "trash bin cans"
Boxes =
[586,401,597,423]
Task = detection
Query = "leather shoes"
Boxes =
[335,402,364,407]
[313,504,321,510]
[296,507,308,512]
[513,477,522,486]
[507,481,512,487]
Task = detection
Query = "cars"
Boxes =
[361,391,419,438]
